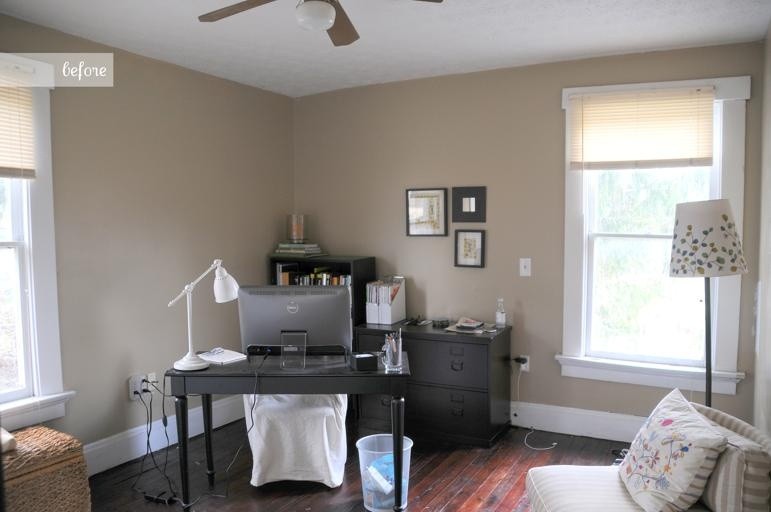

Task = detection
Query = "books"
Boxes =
[274,242,353,309]
[367,280,400,304]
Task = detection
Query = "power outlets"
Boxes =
[127,373,156,401]
[519,354,529,372]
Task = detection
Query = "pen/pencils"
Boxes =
[384,328,402,367]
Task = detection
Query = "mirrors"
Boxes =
[451,186,487,223]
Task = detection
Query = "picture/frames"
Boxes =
[453,228,484,268]
[404,188,448,237]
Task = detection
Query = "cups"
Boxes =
[385,338,404,372]
[288,214,306,243]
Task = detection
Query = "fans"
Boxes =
[198,0,446,48]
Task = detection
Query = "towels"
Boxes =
[243,391,346,488]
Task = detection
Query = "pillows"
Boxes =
[614,387,728,512]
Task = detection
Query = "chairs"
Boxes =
[524,400,770,512]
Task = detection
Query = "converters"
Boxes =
[144,488,176,504]
[515,358,526,364]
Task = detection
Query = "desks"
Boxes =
[165,350,410,512]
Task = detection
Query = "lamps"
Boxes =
[667,199,750,407]
[295,0,336,31]
[167,257,240,371]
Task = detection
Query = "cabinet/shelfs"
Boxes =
[354,320,512,449]
[270,253,376,354]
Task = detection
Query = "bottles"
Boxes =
[495,297,507,328]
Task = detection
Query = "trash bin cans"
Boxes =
[355,433,414,512]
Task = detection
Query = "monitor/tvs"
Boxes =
[238,285,352,355]
